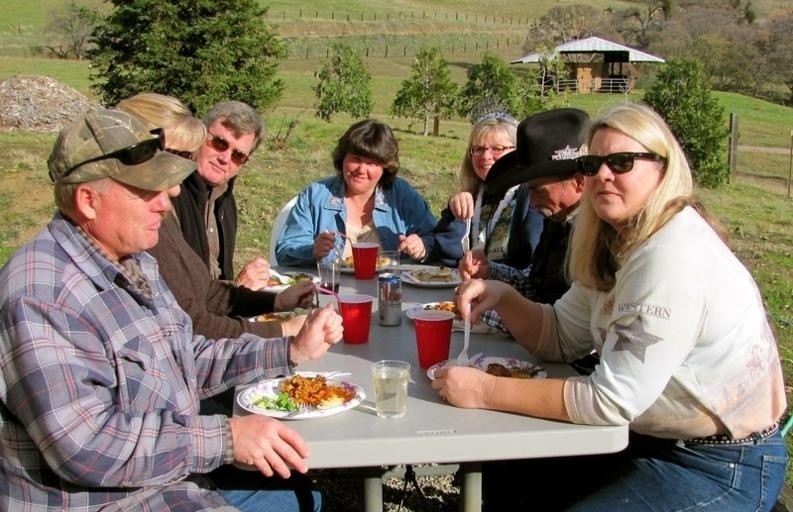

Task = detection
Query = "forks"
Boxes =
[458,302,472,367]
[325,229,348,268]
[461,217,472,256]
[281,403,313,418]
[266,268,295,285]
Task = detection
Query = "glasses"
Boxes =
[63,127,165,178]
[167,147,193,160]
[576,151,667,177]
[471,145,516,155]
[207,130,250,166]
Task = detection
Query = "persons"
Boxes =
[433,112,544,269]
[275,118,439,265]
[459,109,595,337]
[113,93,317,342]
[168,100,271,291]
[432,103,789,512]
[1,108,344,512]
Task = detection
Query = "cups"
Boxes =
[372,360,412,420]
[351,242,380,280]
[414,310,456,369]
[317,262,340,296]
[336,294,374,345]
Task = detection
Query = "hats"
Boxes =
[48,110,199,192]
[483,109,593,192]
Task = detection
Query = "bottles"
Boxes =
[377,275,402,326]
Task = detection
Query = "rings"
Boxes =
[442,398,447,402]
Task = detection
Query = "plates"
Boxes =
[248,311,310,324]
[405,300,474,325]
[263,271,319,292]
[400,267,468,287]
[427,356,547,388]
[236,373,367,420]
[328,254,399,274]
